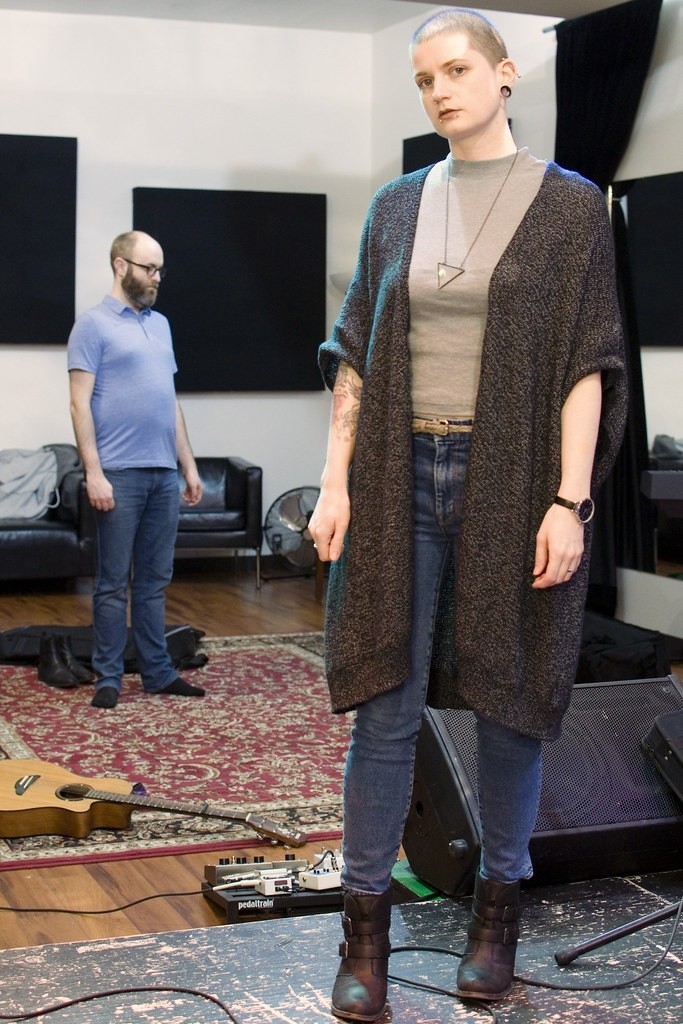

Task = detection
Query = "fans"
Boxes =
[264,486,321,570]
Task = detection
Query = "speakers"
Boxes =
[400,672,683,899]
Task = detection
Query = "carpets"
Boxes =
[0,630,357,872]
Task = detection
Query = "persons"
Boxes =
[307,9,630,1020]
[67,231,206,709]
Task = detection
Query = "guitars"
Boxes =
[0,758,307,850]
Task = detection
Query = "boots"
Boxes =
[456,866,522,1001]
[38,632,96,687]
[331,885,393,1023]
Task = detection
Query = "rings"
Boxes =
[568,568,577,573]
[313,543,317,548]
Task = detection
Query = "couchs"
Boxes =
[0,443,97,580]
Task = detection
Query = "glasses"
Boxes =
[125,258,167,278]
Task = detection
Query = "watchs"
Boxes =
[552,494,595,524]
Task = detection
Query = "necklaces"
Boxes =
[436,149,518,288]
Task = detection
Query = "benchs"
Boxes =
[132,457,262,589]
